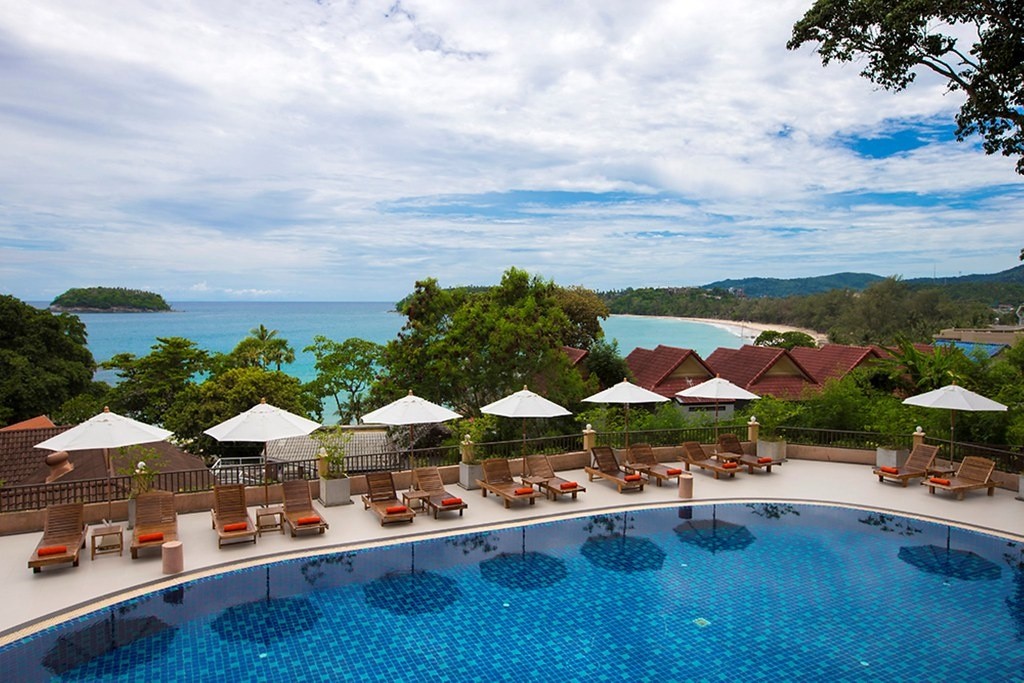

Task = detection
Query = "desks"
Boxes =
[708,452,741,466]
[624,462,651,485]
[522,475,550,500]
[90,525,122,561]
[256,506,286,538]
[927,465,957,479]
[402,491,430,516]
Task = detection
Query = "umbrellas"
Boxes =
[34,406,175,530]
[674,373,761,459]
[479,385,572,484]
[479,525,569,594]
[675,504,756,556]
[582,511,666,573]
[581,377,671,470]
[362,389,463,490]
[365,541,462,615]
[40,604,175,682]
[204,396,322,507]
[898,525,1003,583]
[901,380,1008,477]
[210,565,322,646]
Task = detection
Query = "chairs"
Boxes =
[718,434,782,474]
[129,492,178,560]
[920,455,1004,500]
[211,484,257,550]
[281,479,329,538]
[475,458,542,509]
[27,502,88,573]
[414,466,468,520]
[871,443,941,489]
[676,441,744,479]
[627,444,687,488]
[361,471,416,527]
[527,455,586,502]
[585,446,651,494]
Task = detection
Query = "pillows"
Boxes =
[561,482,577,491]
[930,478,951,486]
[624,475,641,482]
[758,457,772,464]
[138,531,164,544]
[722,462,738,469]
[223,522,247,532]
[297,516,321,525]
[442,498,462,506]
[881,466,899,475]
[667,470,682,475]
[37,545,67,557]
[385,506,408,515]
[514,488,534,495]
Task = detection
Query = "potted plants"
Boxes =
[756,434,786,462]
[309,421,354,508]
[457,459,486,491]
[111,442,170,532]
[876,444,910,468]
